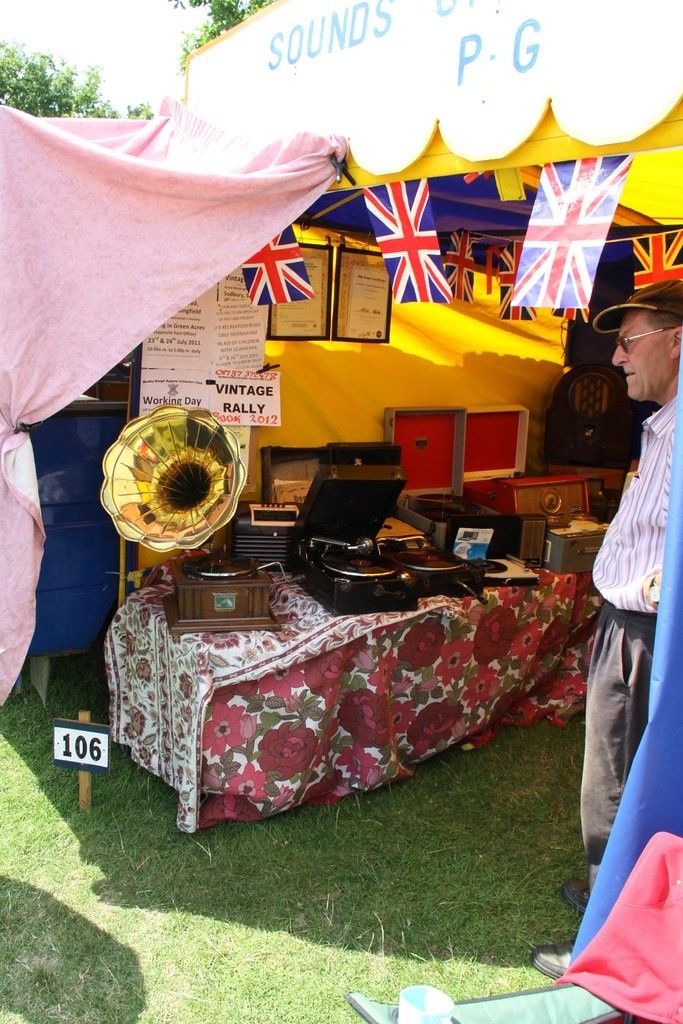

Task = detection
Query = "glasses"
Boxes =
[615,326,676,354]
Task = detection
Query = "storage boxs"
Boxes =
[229,405,608,617]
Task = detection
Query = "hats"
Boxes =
[591,279,683,333]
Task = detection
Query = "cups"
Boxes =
[398,985,452,1024]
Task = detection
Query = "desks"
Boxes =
[104,555,608,834]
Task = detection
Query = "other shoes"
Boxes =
[531,939,575,980]
[561,876,591,915]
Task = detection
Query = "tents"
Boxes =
[0,104,349,712]
[119,0,683,605]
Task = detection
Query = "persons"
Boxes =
[530,278,683,980]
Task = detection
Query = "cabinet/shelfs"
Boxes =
[23,357,131,658]
